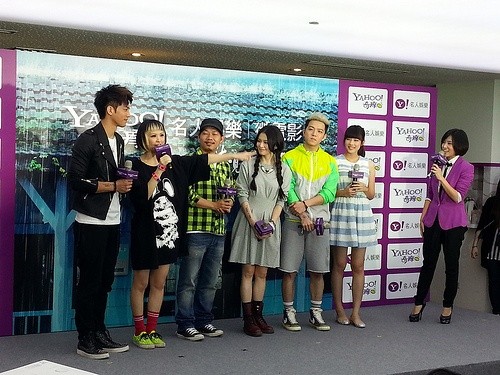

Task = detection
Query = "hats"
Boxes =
[200,118,223,134]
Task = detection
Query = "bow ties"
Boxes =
[445,162,453,168]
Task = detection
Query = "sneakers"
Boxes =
[178,326,204,341]
[93,330,130,353]
[133,331,155,349]
[146,330,166,348]
[76,338,109,360]
[199,323,224,337]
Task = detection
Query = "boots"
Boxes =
[253,301,275,334]
[308,307,331,331]
[241,303,263,336]
[281,308,302,331]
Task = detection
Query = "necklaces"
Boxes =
[260,162,275,173]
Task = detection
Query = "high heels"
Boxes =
[439,306,452,324]
[408,302,426,322]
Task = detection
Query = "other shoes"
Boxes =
[350,315,366,327]
[336,314,350,325]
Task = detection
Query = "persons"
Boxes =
[70,85,133,359]
[123,119,257,349]
[175,117,236,341]
[278,112,340,331]
[470,180,500,314]
[408,129,474,324]
[328,125,376,328]
[234,125,291,337]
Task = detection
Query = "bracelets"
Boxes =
[303,201,308,210]
[152,172,160,182]
[157,163,167,172]
[113,181,117,193]
[472,246,478,248]
[269,219,276,230]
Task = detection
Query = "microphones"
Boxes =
[223,178,232,200]
[429,150,444,179]
[156,143,173,171]
[257,218,269,242]
[298,222,333,230]
[122,160,133,199]
[353,164,360,189]
